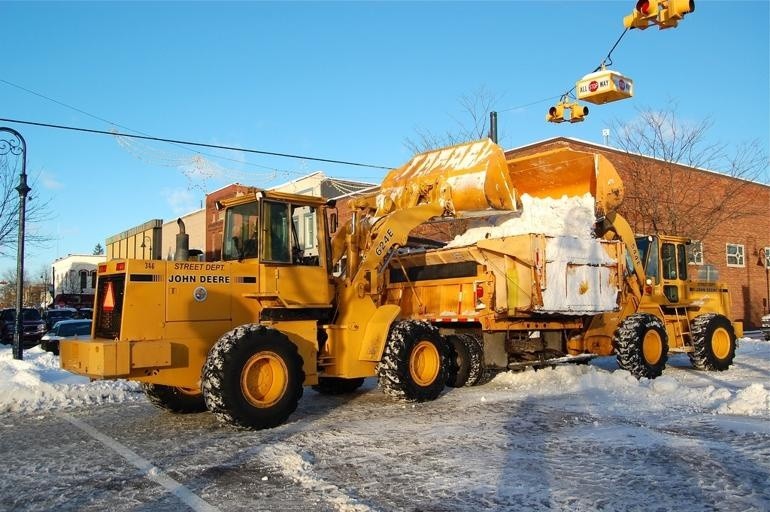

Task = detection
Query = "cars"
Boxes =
[0,301,96,361]
[761,313,770,341]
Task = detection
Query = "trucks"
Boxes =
[383,226,631,388]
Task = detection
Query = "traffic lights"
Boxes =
[622,0,695,29]
[545,103,564,122]
[570,103,589,122]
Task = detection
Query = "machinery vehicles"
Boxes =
[58,134,525,432]
[503,138,746,379]
